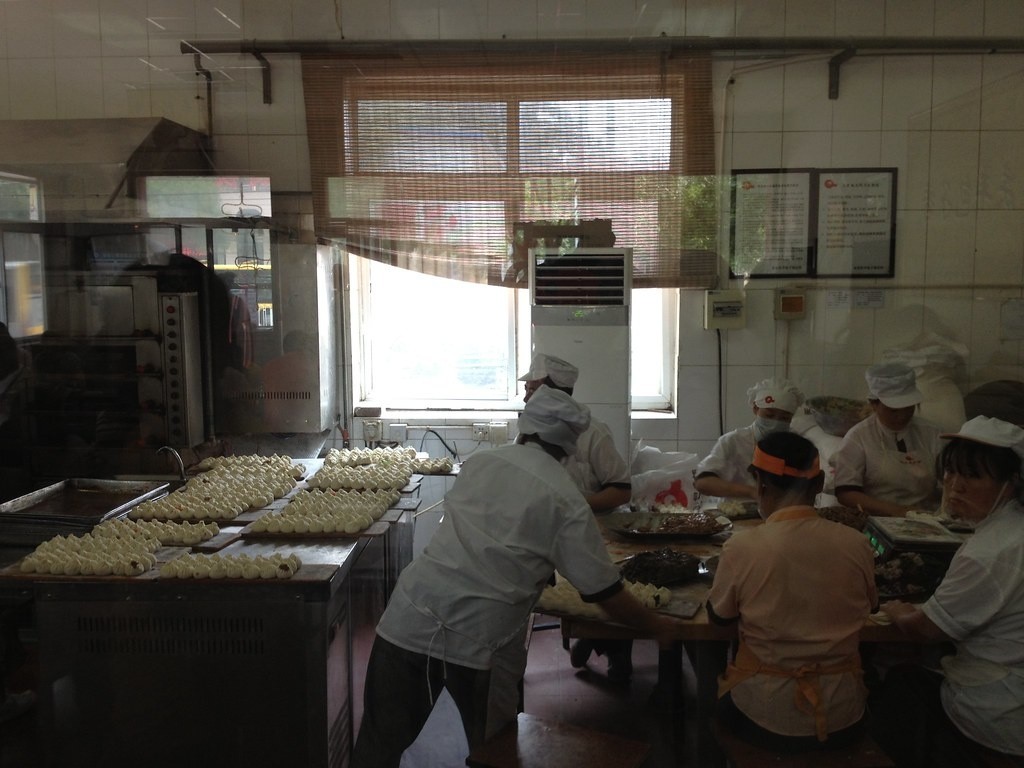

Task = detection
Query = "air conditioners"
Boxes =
[530,247,633,477]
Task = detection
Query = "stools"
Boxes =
[705,718,896,768]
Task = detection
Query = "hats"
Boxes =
[517,384,593,456]
[745,376,806,416]
[865,362,924,410]
[938,414,1024,460]
[517,353,579,388]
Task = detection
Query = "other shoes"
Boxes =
[570,639,593,668]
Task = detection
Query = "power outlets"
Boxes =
[362,420,382,441]
[473,423,489,441]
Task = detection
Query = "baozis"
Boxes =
[717,499,747,517]
[20,446,454,580]
[534,570,671,620]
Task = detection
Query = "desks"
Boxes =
[533,504,948,768]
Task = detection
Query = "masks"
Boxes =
[756,418,791,438]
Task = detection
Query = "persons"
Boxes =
[683,376,805,698]
[825,361,954,519]
[516,354,635,679]
[883,415,1024,767]
[705,429,881,768]
[347,382,686,768]
